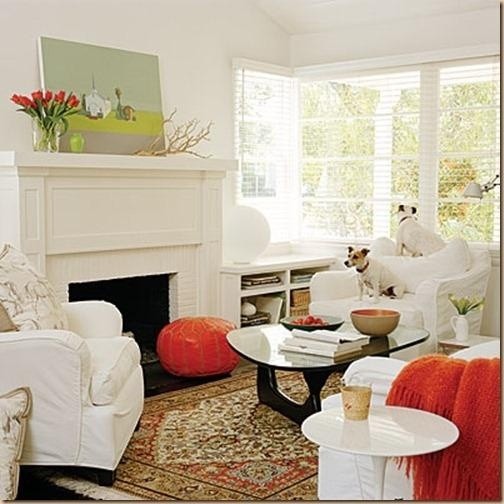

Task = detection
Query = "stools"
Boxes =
[157,315,241,377]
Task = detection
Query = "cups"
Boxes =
[340,384,372,423]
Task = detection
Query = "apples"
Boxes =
[290,315,324,325]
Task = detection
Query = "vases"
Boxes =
[70,132,85,153]
[30,118,60,153]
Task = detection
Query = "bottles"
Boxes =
[69,132,85,153]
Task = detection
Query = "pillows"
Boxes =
[0,244,68,332]
[0,388,30,501]
[376,239,474,293]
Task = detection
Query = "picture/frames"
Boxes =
[37,34,167,157]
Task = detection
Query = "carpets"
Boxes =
[50,366,345,501]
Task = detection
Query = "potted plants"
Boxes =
[449,293,485,340]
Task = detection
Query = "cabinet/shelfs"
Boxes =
[217,252,337,332]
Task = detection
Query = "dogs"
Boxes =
[344,246,406,301]
[394,204,447,257]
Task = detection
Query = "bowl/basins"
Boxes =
[350,308,401,337]
[280,314,345,332]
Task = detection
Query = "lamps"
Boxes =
[462,174,500,200]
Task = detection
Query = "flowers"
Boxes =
[11,90,83,151]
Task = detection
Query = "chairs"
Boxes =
[0,285,146,488]
[317,340,502,502]
[309,239,491,363]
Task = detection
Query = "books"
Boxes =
[289,271,314,278]
[241,311,272,326]
[277,328,370,358]
[291,278,311,283]
[279,350,363,365]
[240,274,283,290]
